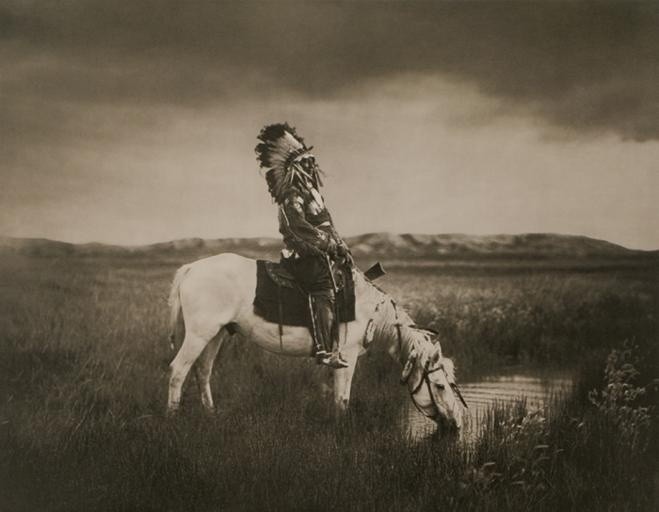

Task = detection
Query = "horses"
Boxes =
[167,253,463,433]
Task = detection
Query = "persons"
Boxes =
[255,122,349,368]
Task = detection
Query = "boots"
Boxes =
[308,295,349,369]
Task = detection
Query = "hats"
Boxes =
[256,123,323,202]
